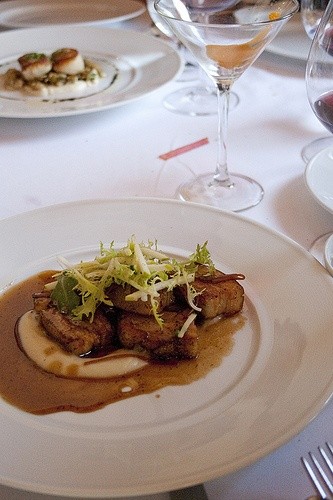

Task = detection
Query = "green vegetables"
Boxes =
[39,234,218,327]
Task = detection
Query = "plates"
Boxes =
[234,0,333,64]
[0,197,333,498]
[0,26,182,119]
[303,144,332,216]
[0,0,148,26]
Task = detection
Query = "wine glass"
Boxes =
[152,0,300,213]
[301,1,333,170]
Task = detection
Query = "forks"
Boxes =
[295,442,333,498]
